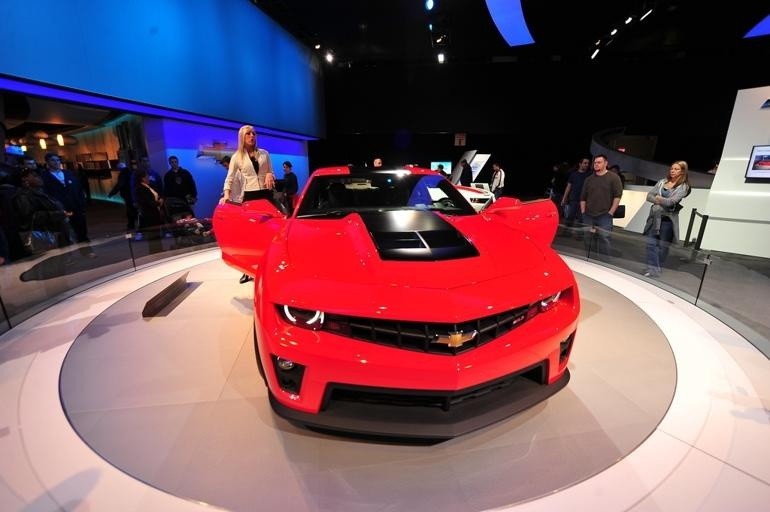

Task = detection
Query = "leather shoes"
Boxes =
[240,274,249,283]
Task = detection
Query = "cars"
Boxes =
[341,168,496,214]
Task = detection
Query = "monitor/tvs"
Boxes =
[744,145,770,183]
[5,145,24,157]
[431,161,452,174]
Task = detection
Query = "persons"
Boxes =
[1,153,97,266]
[105,154,198,254]
[220,156,242,202]
[283,161,298,216]
[641,160,692,280]
[489,162,506,198]
[219,124,276,284]
[460,158,473,188]
[542,154,626,264]
[435,164,448,177]
[373,158,383,167]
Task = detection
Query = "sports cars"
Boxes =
[206,162,582,440]
